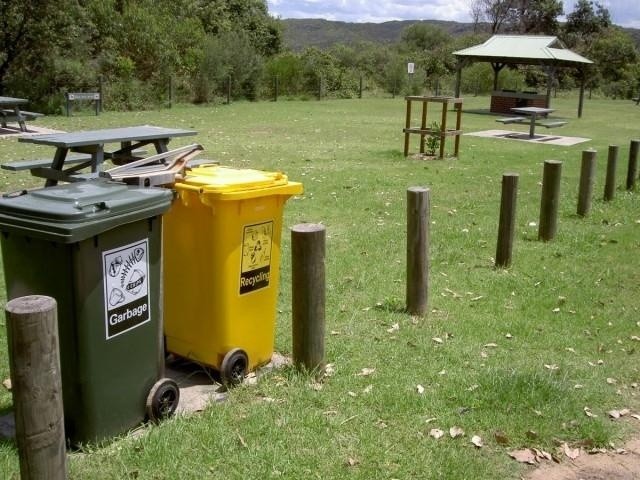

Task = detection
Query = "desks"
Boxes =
[18,124,198,186]
[0,96,29,132]
[510,106,555,137]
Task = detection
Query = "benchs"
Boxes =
[545,121,568,128]
[3,109,44,117]
[1,147,147,172]
[495,117,525,125]
